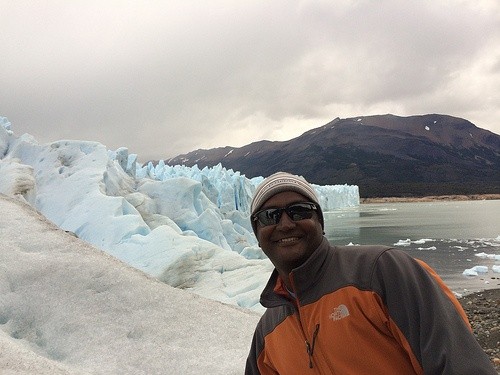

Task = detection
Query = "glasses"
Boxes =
[253,201,319,226]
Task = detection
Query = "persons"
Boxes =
[242,171,499,375]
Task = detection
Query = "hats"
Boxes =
[250,171,324,238]
